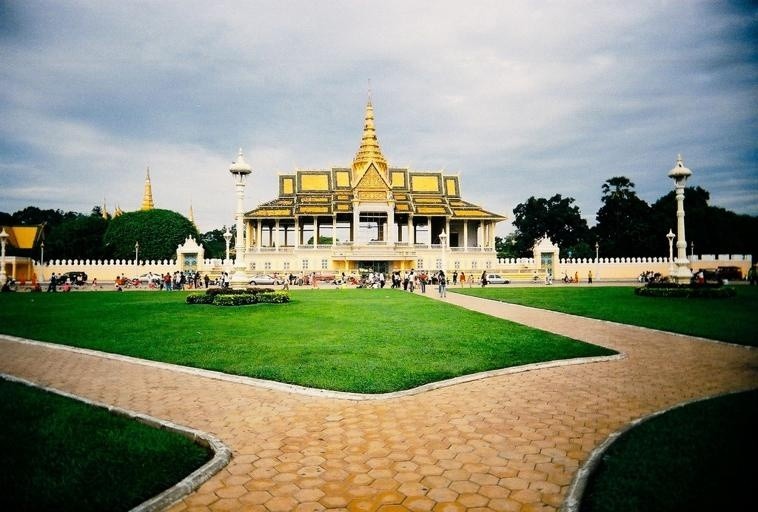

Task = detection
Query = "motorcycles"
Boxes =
[113,277,130,289]
[637,271,655,283]
[561,277,571,284]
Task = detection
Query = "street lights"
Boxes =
[666,227,676,276]
[690,241,694,268]
[134,242,139,279]
[0,228,9,289]
[223,227,232,288]
[668,154,693,285]
[438,228,447,289]
[594,240,599,281]
[38,242,44,281]
[228,145,253,290]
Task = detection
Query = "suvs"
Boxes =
[54,271,88,286]
[746,265,757,285]
[691,269,721,288]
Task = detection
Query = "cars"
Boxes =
[132,272,167,283]
[247,270,450,287]
[477,274,511,285]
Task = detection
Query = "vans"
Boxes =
[714,265,741,281]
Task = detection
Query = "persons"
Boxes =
[7,270,229,292]
[533,271,594,285]
[268,269,489,297]
[696,270,704,283]
[640,268,659,284]
